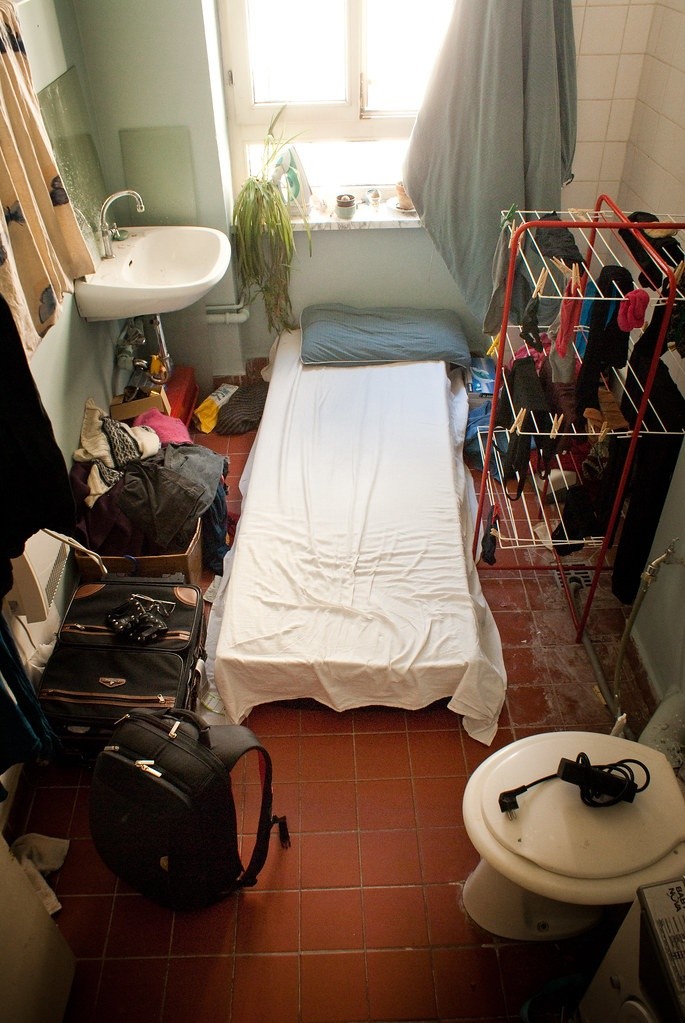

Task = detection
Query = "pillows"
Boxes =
[299,302,472,369]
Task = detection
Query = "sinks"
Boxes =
[75,226,231,323]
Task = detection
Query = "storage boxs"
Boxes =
[108,382,172,429]
[72,516,205,587]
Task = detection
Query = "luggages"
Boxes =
[36,570,204,762]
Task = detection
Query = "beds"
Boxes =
[213,327,508,746]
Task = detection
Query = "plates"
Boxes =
[387,196,416,212]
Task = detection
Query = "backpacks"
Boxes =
[88,706,272,913]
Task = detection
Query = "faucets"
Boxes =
[645,538,685,578]
[100,190,145,259]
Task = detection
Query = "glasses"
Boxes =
[129,593,177,617]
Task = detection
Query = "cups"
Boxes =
[335,206,355,220]
[337,195,355,207]
[368,192,381,207]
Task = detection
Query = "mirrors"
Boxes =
[32,63,118,266]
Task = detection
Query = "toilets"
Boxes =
[461,731,685,940]
